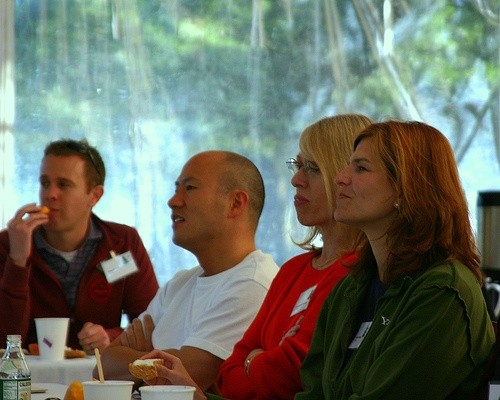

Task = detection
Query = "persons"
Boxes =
[130,121,496,400]
[215,113,380,400]
[89,149,280,385]
[0,136,159,355]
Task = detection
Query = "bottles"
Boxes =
[0,335,32,400]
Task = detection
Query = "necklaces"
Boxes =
[314,256,341,267]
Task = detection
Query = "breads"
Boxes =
[128,359,165,382]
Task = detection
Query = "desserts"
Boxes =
[29,343,39,355]
[29,206,51,215]
[63,380,85,400]
[64,349,86,359]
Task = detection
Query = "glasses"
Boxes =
[286,158,324,178]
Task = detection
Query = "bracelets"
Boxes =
[244,351,262,376]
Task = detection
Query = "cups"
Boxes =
[35,318,69,361]
[81,380,134,400]
[138,384,196,400]
